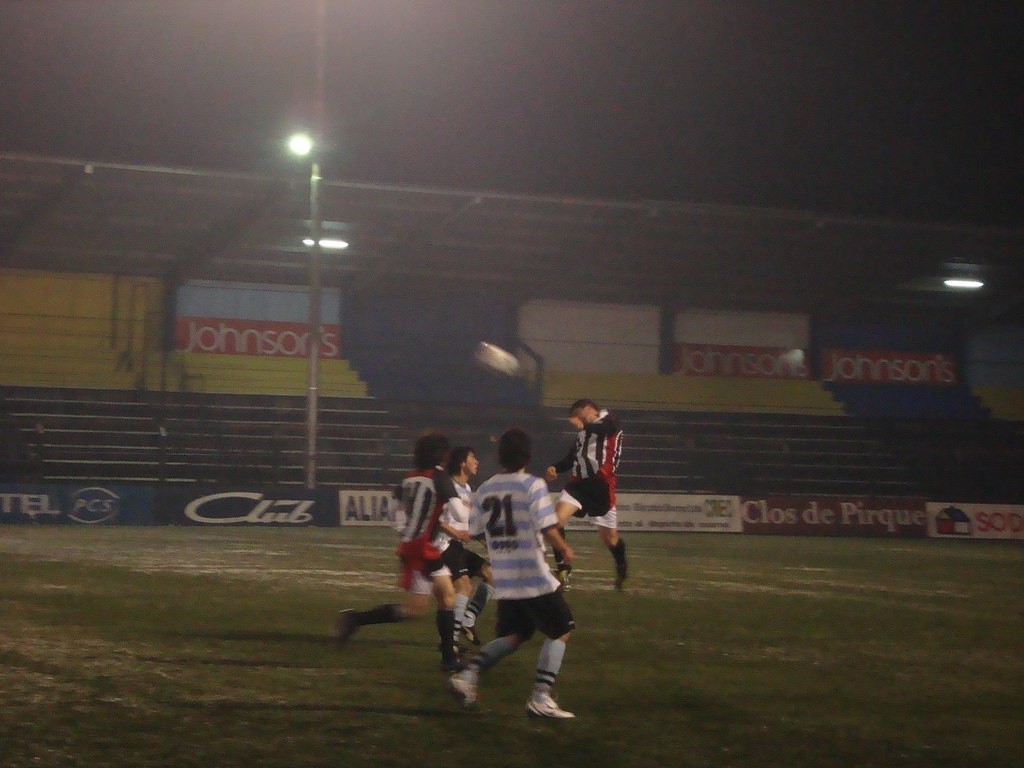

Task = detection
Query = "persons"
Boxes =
[545,398,630,594]
[336,433,494,675]
[447,429,576,720]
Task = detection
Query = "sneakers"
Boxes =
[440,660,465,672]
[525,697,575,719]
[449,673,479,710]
[461,623,481,646]
[338,609,354,647]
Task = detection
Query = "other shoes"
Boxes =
[615,566,625,587]
[554,563,570,584]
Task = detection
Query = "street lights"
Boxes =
[288,131,324,492]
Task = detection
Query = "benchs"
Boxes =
[0,149,1024,504]
